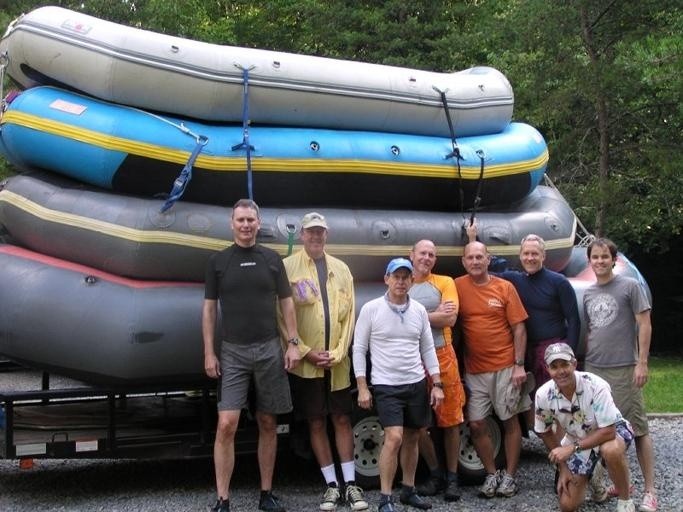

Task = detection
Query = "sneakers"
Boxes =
[318,469,519,506]
[638,492,658,511]
[215,497,229,506]
[618,498,636,512]
[591,477,609,505]
[607,482,635,497]
[258,490,280,507]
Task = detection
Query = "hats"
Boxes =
[544,341,575,365]
[385,258,415,277]
[300,212,330,232]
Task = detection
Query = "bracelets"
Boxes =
[432,381,444,388]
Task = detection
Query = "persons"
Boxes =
[533,342,636,512]
[453,241,529,497]
[468,217,579,494]
[202,200,300,512]
[409,239,466,502]
[276,211,369,512]
[580,237,657,512]
[352,259,446,511]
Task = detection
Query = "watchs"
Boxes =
[574,440,582,454]
[288,338,298,345]
[514,359,524,366]
